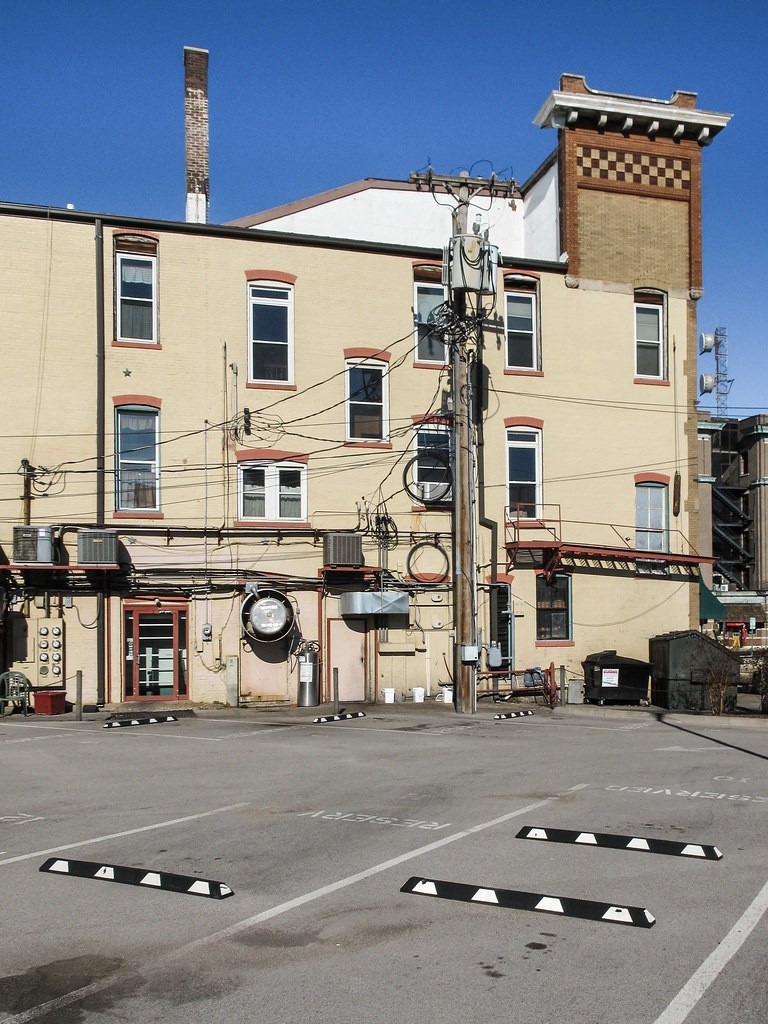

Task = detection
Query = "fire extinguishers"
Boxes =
[296,638,320,707]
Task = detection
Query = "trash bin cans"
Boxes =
[567,678,585,704]
[580,649,653,707]
[648,628,745,716]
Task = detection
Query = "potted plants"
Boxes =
[743,647,768,715]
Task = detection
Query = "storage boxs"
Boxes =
[33,691,68,715]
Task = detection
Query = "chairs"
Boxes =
[0,671,28,717]
